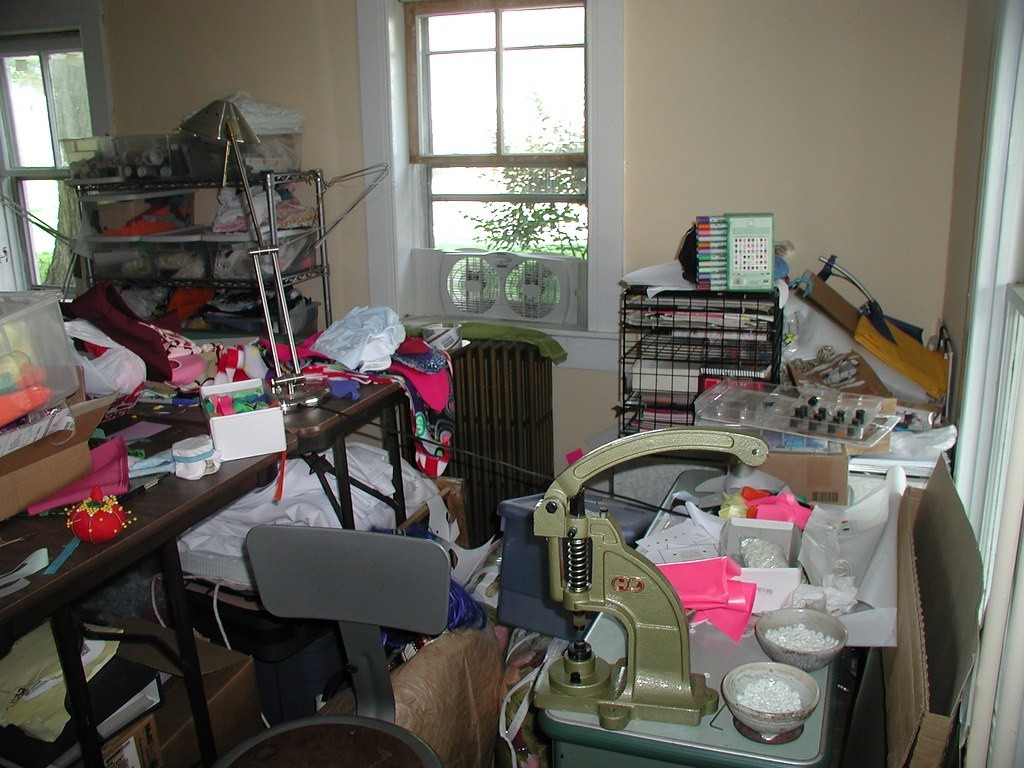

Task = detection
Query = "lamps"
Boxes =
[180,100,331,412]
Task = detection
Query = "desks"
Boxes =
[134,370,416,534]
[539,470,838,768]
[0,374,299,768]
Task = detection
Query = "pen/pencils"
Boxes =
[138,397,197,404]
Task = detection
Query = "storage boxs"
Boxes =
[92,244,187,279]
[0,604,347,768]
[97,188,236,231]
[199,378,287,461]
[728,441,849,506]
[725,518,802,612]
[0,290,120,521]
[58,134,198,176]
[881,454,985,768]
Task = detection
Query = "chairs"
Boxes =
[213,525,451,768]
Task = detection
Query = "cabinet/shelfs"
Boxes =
[65,169,334,347]
[612,284,784,435]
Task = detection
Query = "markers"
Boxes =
[697,215,727,290]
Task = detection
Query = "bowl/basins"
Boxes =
[755,608,848,673]
[722,662,820,734]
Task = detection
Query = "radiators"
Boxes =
[428,339,554,546]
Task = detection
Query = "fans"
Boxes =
[411,248,581,326]
